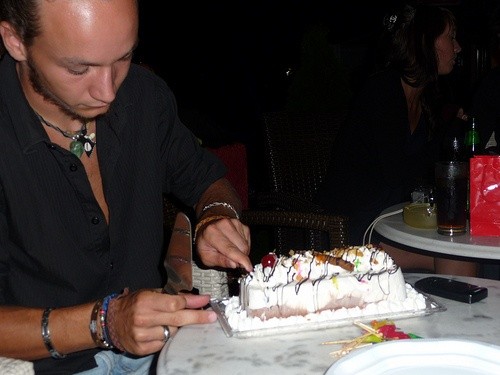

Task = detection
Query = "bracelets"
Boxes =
[202,201,240,220]
[41,307,67,359]
[100,293,121,354]
[89,299,111,349]
[193,215,234,243]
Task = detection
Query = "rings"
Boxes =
[162,324,170,343]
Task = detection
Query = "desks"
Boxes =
[374,201,500,277]
[157,273,500,375]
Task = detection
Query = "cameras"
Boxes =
[411,187,436,207]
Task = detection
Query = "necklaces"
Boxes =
[33,108,96,158]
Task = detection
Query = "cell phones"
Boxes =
[415,277,488,304]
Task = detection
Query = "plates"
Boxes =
[325,336,500,375]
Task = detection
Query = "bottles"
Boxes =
[462,115,483,219]
[434,138,468,237]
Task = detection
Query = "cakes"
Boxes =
[224,244,426,331]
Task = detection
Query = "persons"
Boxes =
[0,0,252,363]
[321,2,479,282]
